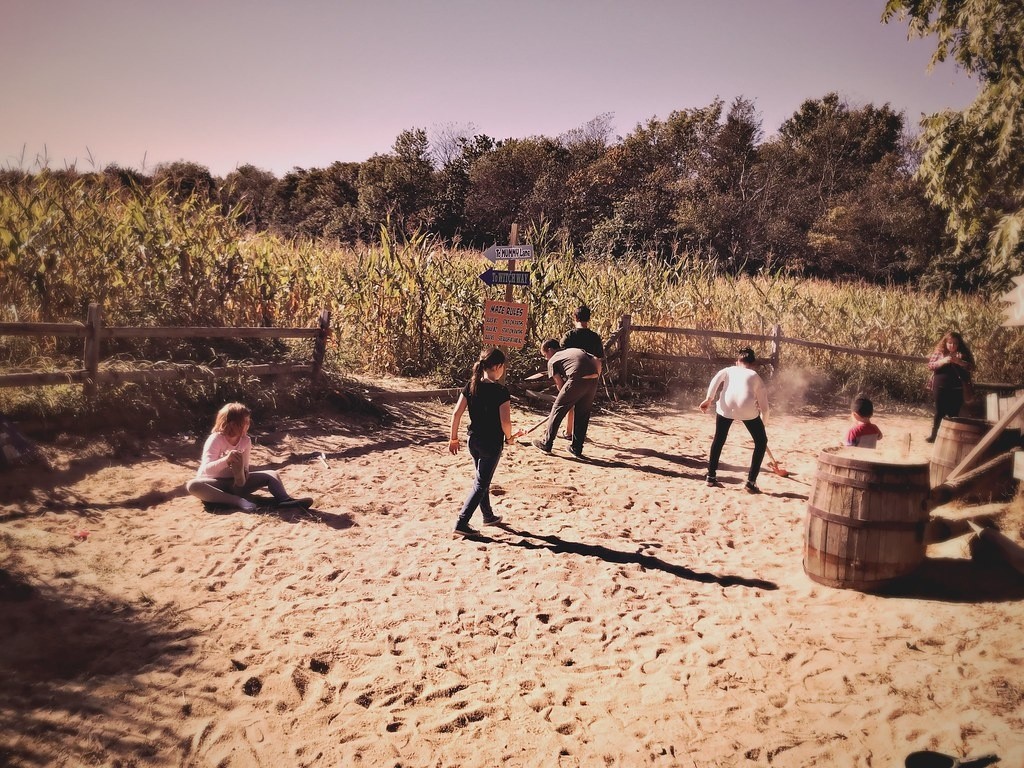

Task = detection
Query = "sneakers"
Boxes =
[532,439,553,454]
[566,444,581,458]
[454,523,480,535]
[743,481,763,494]
[483,515,502,526]
[705,475,718,486]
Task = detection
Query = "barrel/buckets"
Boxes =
[801,444,931,590]
[926,416,1015,503]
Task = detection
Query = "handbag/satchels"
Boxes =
[961,371,975,401]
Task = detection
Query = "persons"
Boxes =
[699,348,769,492]
[449,348,513,536]
[925,332,976,443]
[848,398,882,448]
[557,306,604,438]
[532,338,601,457]
[188,403,313,511]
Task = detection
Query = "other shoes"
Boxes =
[232,453,246,488]
[925,438,934,443]
[278,498,314,509]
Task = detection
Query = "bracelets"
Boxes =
[449,439,459,443]
[506,436,512,440]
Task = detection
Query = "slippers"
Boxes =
[556,430,572,440]
